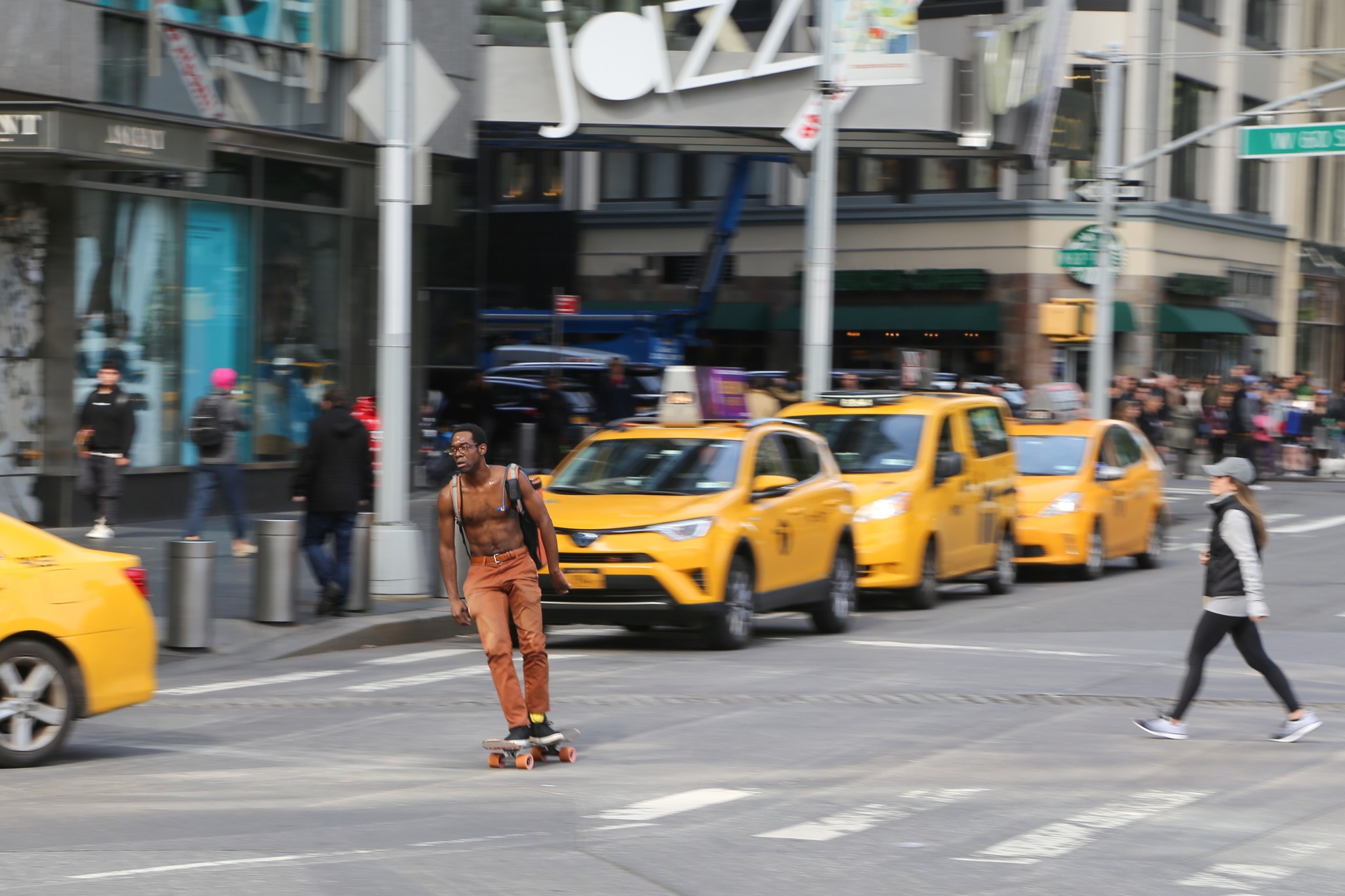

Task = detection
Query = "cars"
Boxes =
[0,515,158,768]
[769,393,1018,613]
[1006,417,1164,584]
[537,409,860,651]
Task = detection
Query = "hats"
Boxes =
[1200,457,1257,485]
[211,369,238,391]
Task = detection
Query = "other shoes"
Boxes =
[86,525,115,539]
[232,545,257,557]
[316,582,342,614]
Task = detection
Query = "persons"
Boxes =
[437,423,569,747]
[1104,360,1329,478]
[288,385,376,616]
[1133,456,1322,743]
[182,369,258,558]
[73,364,137,539]
[533,346,865,471]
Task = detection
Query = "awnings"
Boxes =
[1114,301,1138,333]
[1157,305,1278,337]
[583,298,1004,336]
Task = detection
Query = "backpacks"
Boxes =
[192,406,223,450]
[450,463,548,570]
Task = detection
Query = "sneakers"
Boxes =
[504,725,531,746]
[530,724,564,744]
[1132,714,1189,740]
[1269,710,1322,743]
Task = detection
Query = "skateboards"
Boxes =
[482,726,580,769]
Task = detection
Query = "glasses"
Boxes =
[448,443,478,455]
